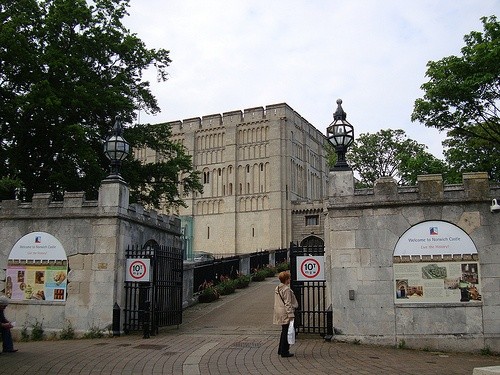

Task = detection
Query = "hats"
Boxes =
[0,295,9,305]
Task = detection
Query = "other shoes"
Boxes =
[278,351,294,357]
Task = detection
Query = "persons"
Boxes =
[273,272,298,357]
[0,294,18,352]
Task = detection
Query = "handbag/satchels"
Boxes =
[294,310,300,326]
[287,318,295,344]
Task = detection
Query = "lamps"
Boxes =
[102,116,126,185]
[326,99,355,171]
